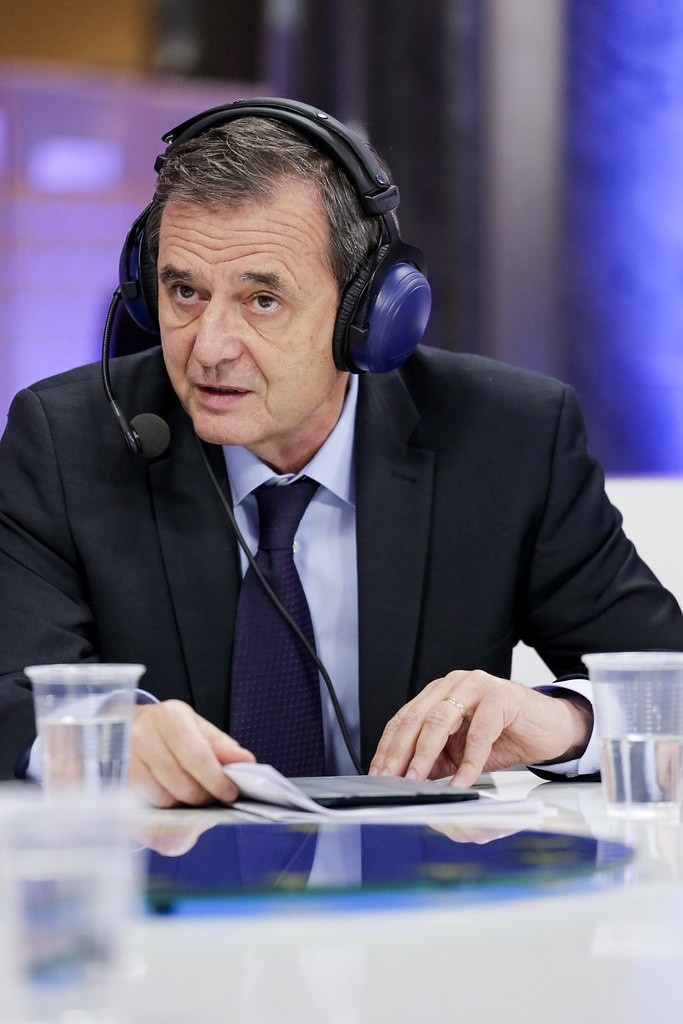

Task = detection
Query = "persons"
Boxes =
[1,100,682,807]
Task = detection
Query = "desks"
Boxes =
[0,785,683,1024]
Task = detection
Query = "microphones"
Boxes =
[101,282,170,461]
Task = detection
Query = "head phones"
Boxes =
[118,95,432,373]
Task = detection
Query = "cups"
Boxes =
[25,663,147,803]
[580,651,683,820]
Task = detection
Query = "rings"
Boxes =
[443,697,466,716]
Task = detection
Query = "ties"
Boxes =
[225,473,328,779]
